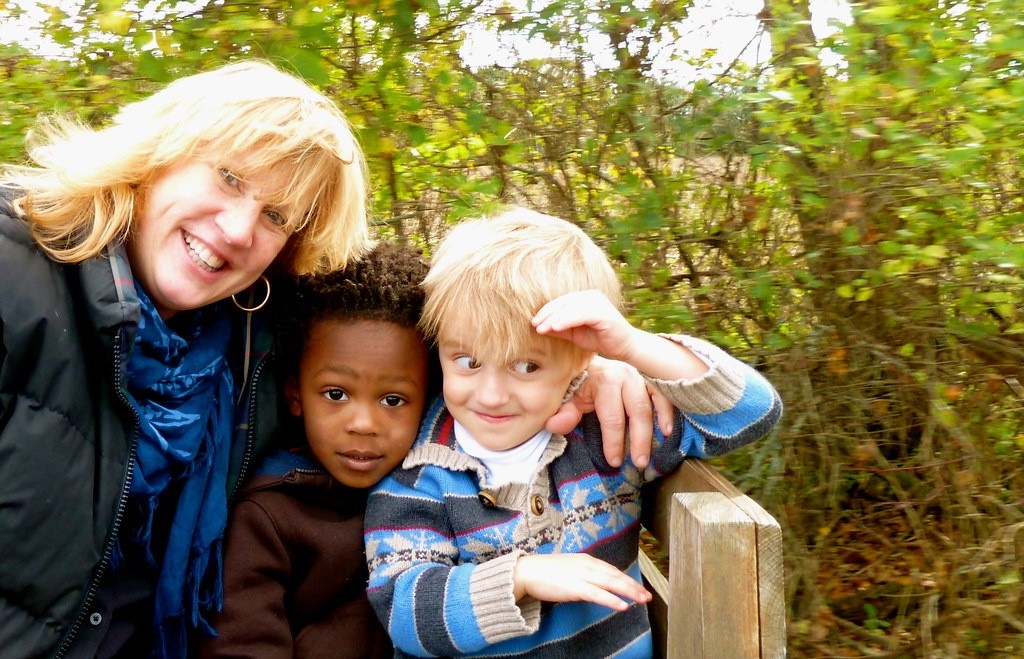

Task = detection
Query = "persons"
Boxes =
[0,56,673,659]
[362,208,784,658]
[199,240,442,659]
